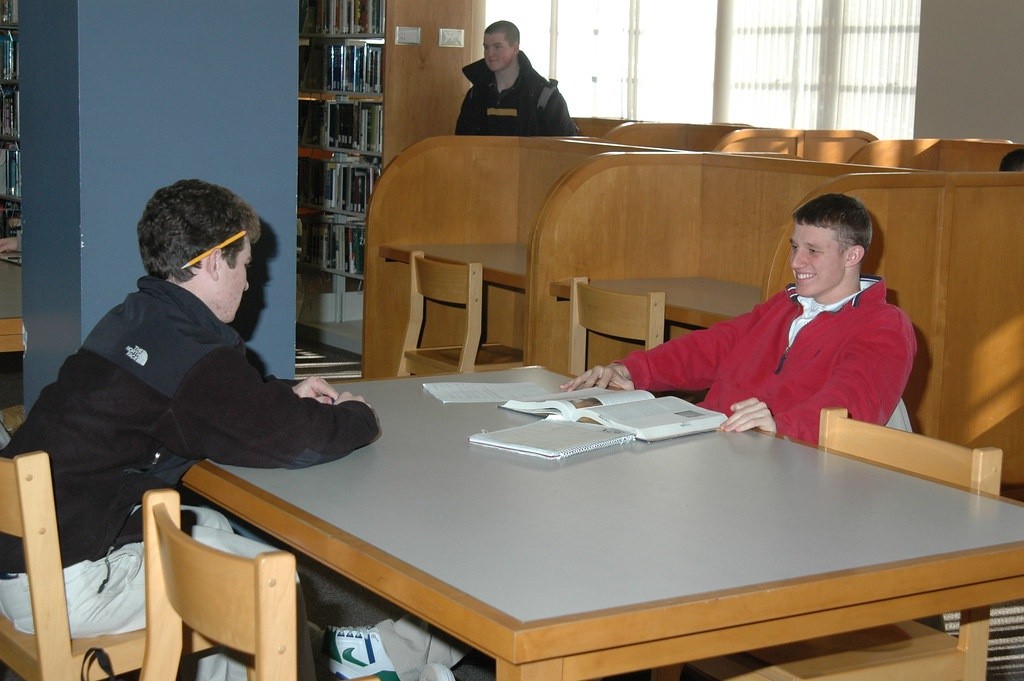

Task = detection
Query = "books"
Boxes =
[500,387,729,442]
[467,414,636,459]
[0,0,22,239]
[298,0,386,293]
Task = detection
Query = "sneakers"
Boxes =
[305,618,400,681]
[420,663,455,681]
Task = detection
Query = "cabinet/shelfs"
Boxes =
[0,19,23,265]
[298,0,486,355]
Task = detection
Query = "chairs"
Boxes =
[567,277,666,376]
[0,450,219,681]
[651,405,1003,681]
[392,250,523,379]
[139,489,299,681]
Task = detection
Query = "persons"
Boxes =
[1000,149,1024,172]
[0,230,22,253]
[454,20,575,137]
[310,194,918,681]
[0,179,397,681]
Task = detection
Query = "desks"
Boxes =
[363,117,1023,487]
[183,365,1023,681]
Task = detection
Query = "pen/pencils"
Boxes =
[4,257,22,259]
[180,230,248,269]
